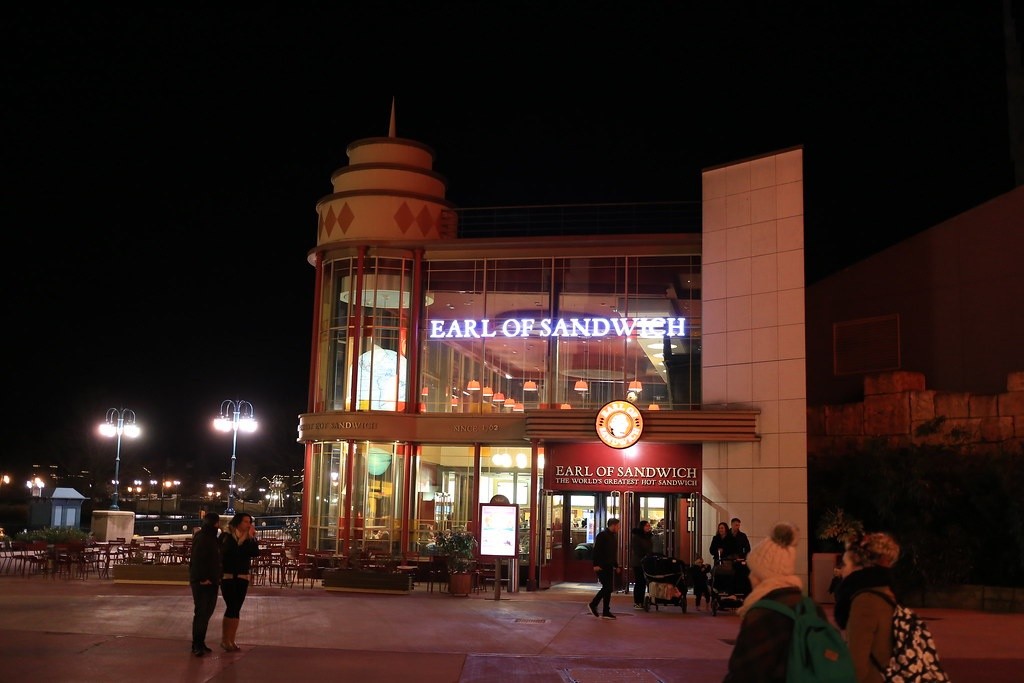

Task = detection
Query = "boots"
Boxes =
[230,618,240,651]
[220,616,234,652]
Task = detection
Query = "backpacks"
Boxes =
[751,597,857,683]
[860,587,949,683]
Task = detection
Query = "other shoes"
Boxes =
[191,647,205,657]
[201,643,212,653]
[696,606,701,611]
[706,603,711,611]
[633,602,646,609]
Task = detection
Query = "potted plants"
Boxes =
[446,548,475,594]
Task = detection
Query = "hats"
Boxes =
[857,533,899,567]
[748,523,799,582]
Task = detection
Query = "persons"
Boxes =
[719,521,841,683]
[189,512,229,655]
[842,533,899,683]
[631,521,654,609]
[690,553,712,611]
[588,518,620,619]
[827,535,858,628]
[709,518,751,565]
[220,513,261,651]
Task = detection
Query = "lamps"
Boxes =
[418,337,660,412]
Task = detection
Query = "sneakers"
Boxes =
[588,602,599,617]
[603,614,616,619]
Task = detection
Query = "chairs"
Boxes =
[397,552,420,586]
[427,555,450,595]
[477,553,496,595]
[0,538,320,590]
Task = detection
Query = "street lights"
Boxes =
[213,399,258,533]
[150,480,158,493]
[173,480,181,511]
[259,488,266,516]
[206,482,214,492]
[90,407,140,544]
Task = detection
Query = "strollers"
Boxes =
[709,557,754,616]
[640,551,688,614]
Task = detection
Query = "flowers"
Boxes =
[433,528,478,555]
